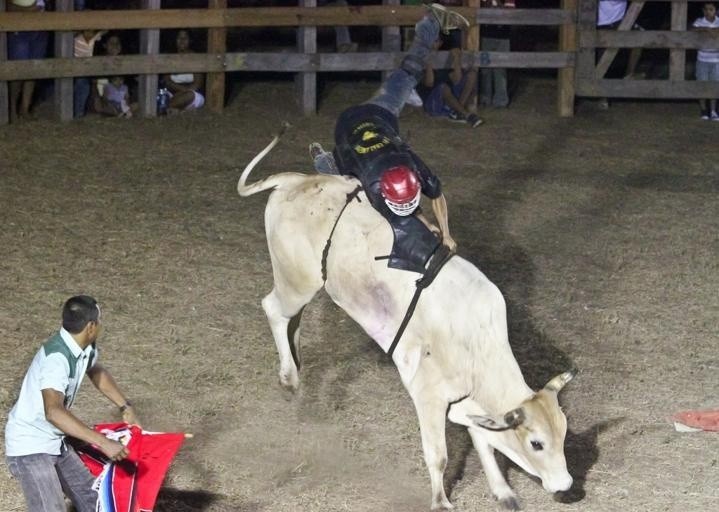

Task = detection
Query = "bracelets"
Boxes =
[120,401,132,414]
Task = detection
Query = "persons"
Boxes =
[693,1,718,121]
[8,1,48,121]
[74,28,132,118]
[417,39,483,128]
[158,30,204,114]
[4,295,143,511]
[597,1,640,79]
[308,3,472,252]
[480,1,514,107]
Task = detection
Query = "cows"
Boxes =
[234,120,579,512]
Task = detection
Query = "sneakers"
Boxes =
[701,111,719,121]
[447,112,481,128]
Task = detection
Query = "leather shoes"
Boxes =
[432,4,469,28]
[309,144,323,158]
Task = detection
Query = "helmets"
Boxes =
[380,166,421,217]
